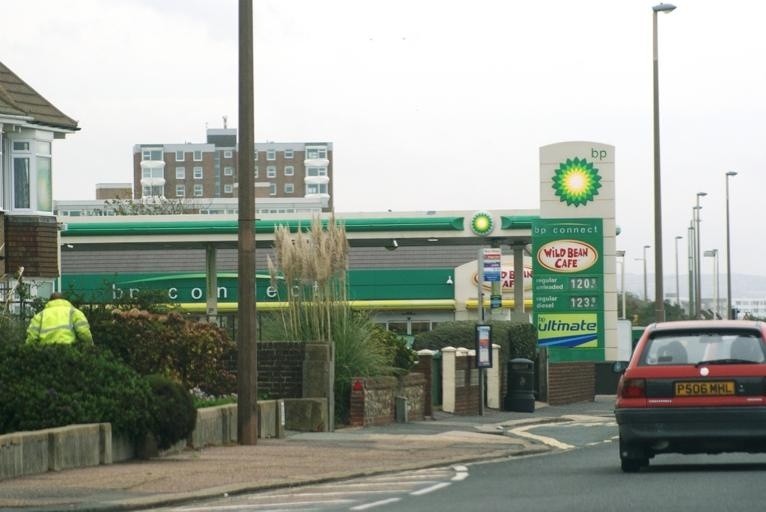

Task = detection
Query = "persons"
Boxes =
[24,291,95,346]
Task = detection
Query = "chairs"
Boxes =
[656,335,761,364]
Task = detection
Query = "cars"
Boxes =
[614,319,766,472]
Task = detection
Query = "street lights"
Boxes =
[643,2,738,322]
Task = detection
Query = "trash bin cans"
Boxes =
[505,358,535,412]
